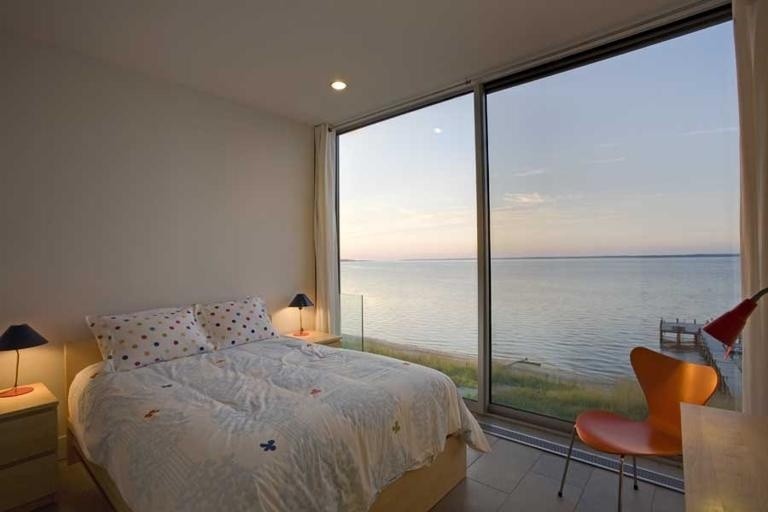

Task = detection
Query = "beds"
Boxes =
[61,336,467,511]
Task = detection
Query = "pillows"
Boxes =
[85,295,279,374]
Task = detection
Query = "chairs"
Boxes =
[558,345,720,512]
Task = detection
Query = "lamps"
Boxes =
[0,323,48,398]
[703,287,768,347]
[287,293,314,336]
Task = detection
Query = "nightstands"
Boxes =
[287,331,343,348]
[0,382,60,512]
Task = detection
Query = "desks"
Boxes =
[678,401,768,512]
